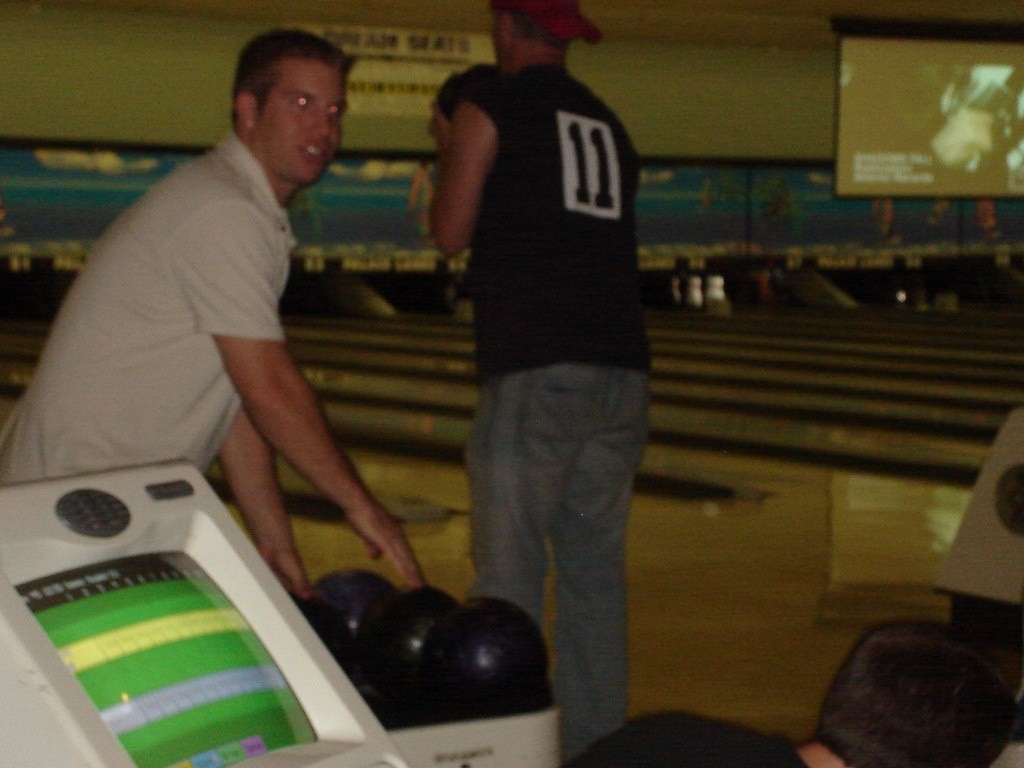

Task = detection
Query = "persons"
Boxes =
[561,618,1016,768]
[423,1,647,768]
[837,57,1011,193]
[0,29,424,595]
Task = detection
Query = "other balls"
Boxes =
[439,62,504,121]
[291,562,552,719]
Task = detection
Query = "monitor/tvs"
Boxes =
[16,550,318,768]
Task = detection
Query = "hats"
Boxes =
[490,0,601,44]
